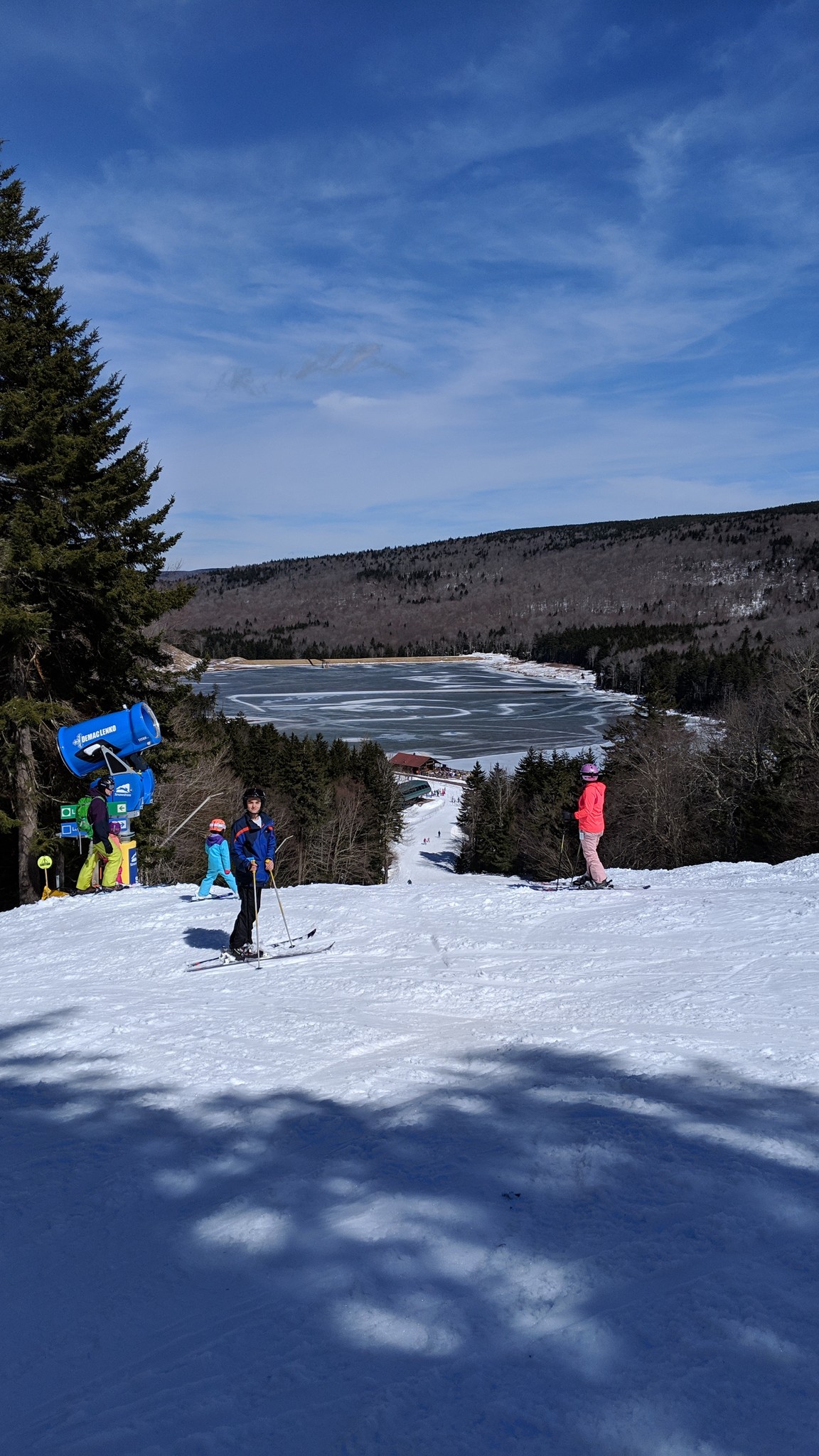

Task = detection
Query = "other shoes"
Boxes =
[578,878,606,889]
[573,871,591,886]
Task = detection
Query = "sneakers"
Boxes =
[241,942,264,958]
[116,882,123,886]
[198,893,218,900]
[69,881,129,897]
[231,946,249,960]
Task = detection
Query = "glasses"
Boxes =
[98,780,114,790]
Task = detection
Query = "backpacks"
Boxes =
[75,795,105,840]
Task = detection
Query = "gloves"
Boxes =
[562,811,575,822]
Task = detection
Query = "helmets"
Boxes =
[209,819,226,834]
[98,776,115,797]
[580,763,599,781]
[108,821,121,836]
[242,788,266,802]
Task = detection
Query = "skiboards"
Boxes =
[185,929,337,974]
[526,879,650,891]
[189,892,234,902]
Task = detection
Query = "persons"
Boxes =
[196,818,240,899]
[72,776,125,895]
[423,838,427,845]
[431,788,445,797]
[100,820,129,890]
[407,878,412,886]
[438,830,441,838]
[562,763,613,888]
[228,787,278,959]
[427,837,430,842]
[451,796,459,803]
